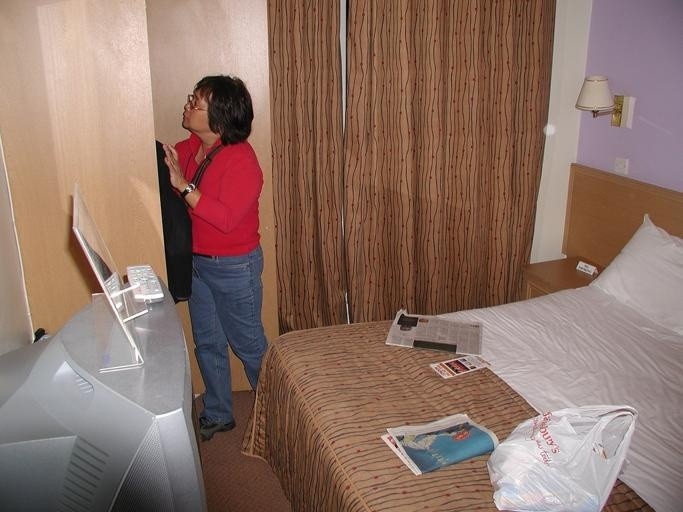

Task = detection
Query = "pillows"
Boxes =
[588,213,682,333]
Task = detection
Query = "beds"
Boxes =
[265,285,682,511]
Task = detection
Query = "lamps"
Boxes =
[575,75,636,129]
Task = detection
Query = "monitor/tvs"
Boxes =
[0,276,209,512]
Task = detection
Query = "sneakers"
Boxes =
[199,416,235,441]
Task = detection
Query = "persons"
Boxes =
[163,74,269,441]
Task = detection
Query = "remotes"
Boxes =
[126,264,164,305]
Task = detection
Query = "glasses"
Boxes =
[188,95,208,112]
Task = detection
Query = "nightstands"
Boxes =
[521,257,604,300]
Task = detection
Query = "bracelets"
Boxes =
[181,183,196,198]
